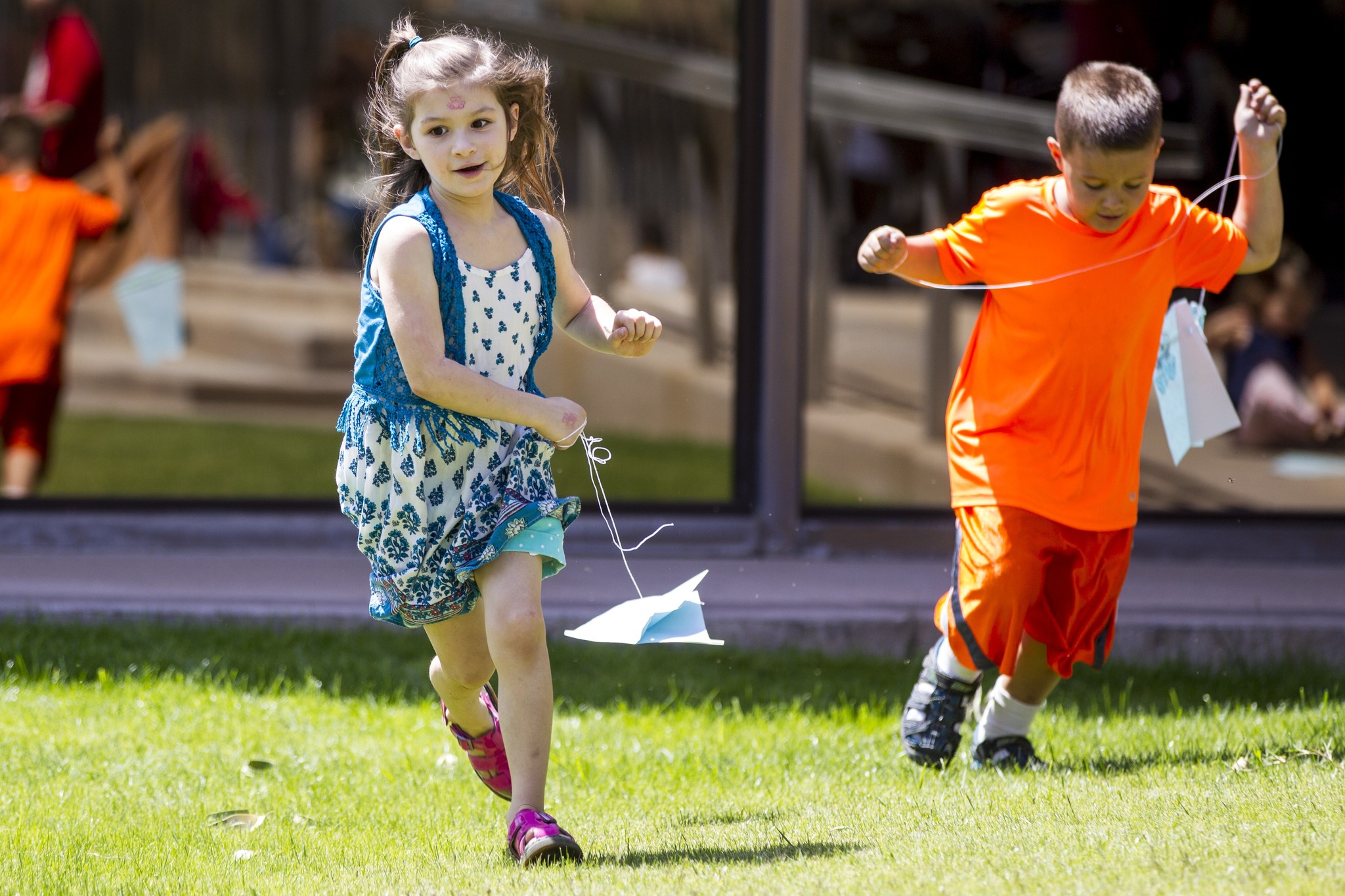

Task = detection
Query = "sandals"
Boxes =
[970,735,1048,774]
[507,808,584,867]
[441,678,513,802]
[900,646,981,773]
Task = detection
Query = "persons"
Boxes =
[854,60,1287,772]
[3,2,366,367]
[330,18,666,873]
[0,109,132,502]
[1199,247,1345,452]
